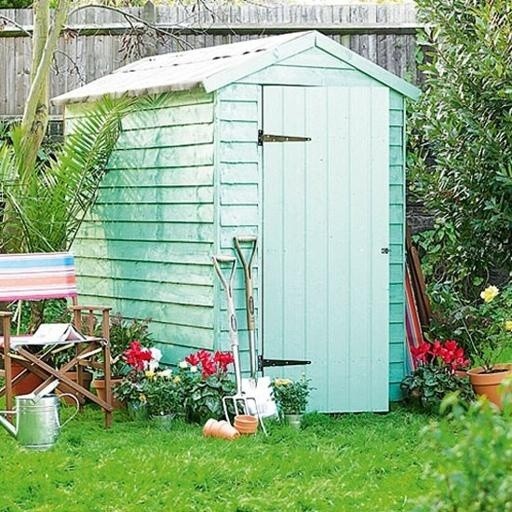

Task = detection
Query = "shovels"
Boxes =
[232,236,281,424]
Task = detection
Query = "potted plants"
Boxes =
[1,88,151,416]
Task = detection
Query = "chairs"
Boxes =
[0,251,114,428]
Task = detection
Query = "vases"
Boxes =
[211,420,241,442]
[284,414,305,429]
[466,363,512,416]
[233,415,259,435]
[201,417,219,436]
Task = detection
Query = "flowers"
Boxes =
[440,283,511,372]
[267,374,318,415]
[114,341,244,418]
